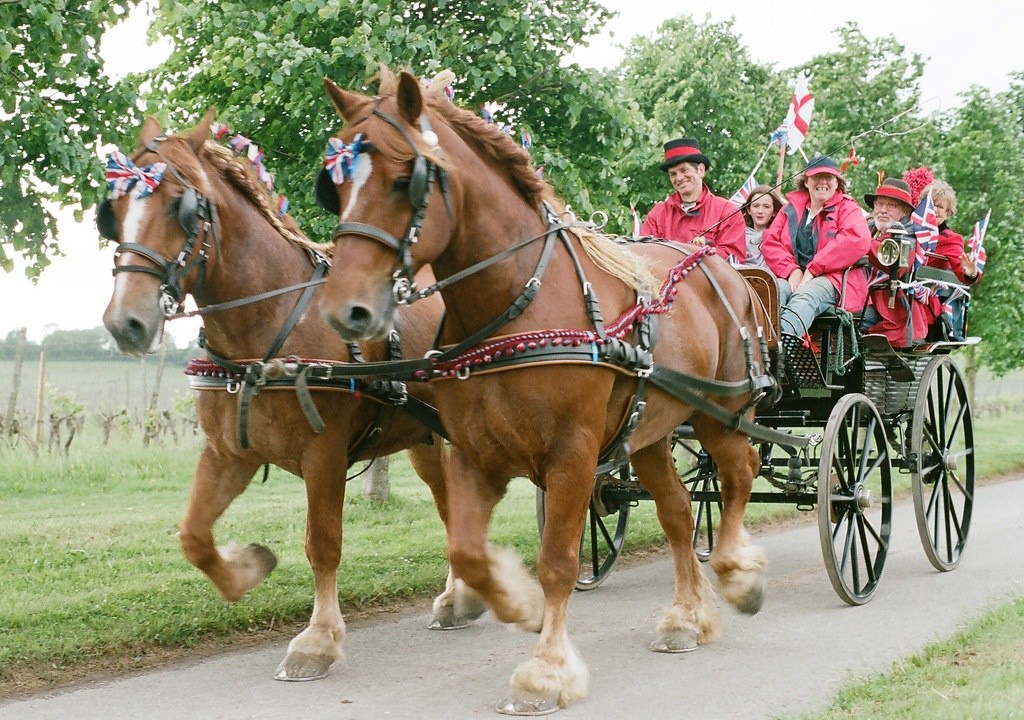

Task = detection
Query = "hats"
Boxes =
[805,156,839,176]
[658,138,710,173]
[864,178,916,215]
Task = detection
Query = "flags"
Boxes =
[730,177,759,207]
[911,194,940,269]
[968,220,989,275]
[785,79,815,154]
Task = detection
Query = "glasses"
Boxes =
[808,173,837,182]
[873,199,908,211]
[934,202,948,213]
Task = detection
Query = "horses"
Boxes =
[95,61,770,716]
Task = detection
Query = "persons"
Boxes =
[916,179,977,328]
[637,138,745,263]
[742,185,788,280]
[862,178,927,348]
[762,156,871,353]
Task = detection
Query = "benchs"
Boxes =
[755,257,982,427]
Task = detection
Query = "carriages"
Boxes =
[96,64,983,717]
